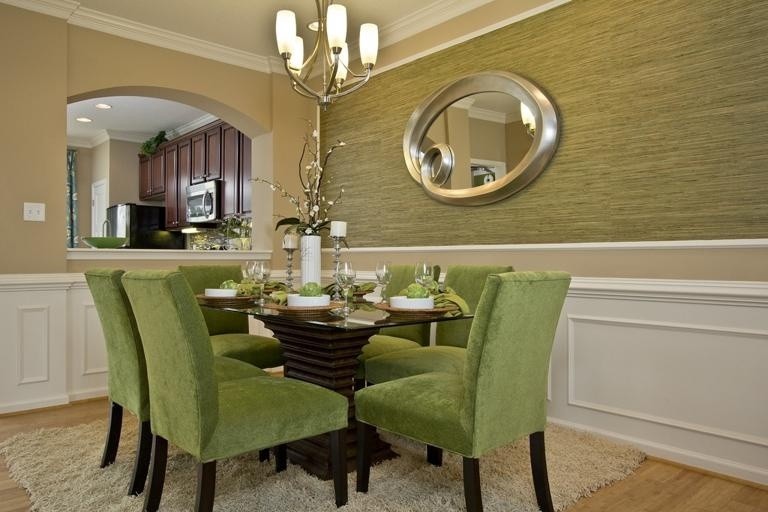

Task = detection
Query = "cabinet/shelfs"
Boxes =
[191,126,221,184]
[139,149,165,198]
[165,138,192,228]
[221,123,251,216]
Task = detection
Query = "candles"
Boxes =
[281,233,298,249]
[330,220,348,237]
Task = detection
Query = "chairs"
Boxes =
[354,261,441,391]
[121,268,349,512]
[364,262,514,467]
[177,263,286,370]
[84,266,272,497]
[354,271,572,512]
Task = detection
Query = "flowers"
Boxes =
[250,116,353,249]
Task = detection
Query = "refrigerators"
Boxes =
[101,202,164,246]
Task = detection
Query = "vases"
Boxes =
[300,236,323,295]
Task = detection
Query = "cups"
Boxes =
[414,261,436,284]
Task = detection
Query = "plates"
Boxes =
[263,302,346,314]
[375,302,460,320]
[196,292,253,307]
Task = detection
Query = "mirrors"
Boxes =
[401,69,563,207]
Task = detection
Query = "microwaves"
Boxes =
[184,179,223,222]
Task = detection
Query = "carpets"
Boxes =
[0,414,648,512]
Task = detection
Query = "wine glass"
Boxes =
[376,260,393,307]
[333,260,357,319]
[243,259,269,303]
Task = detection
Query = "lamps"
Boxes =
[275,0,379,113]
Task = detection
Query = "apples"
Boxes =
[251,287,260,294]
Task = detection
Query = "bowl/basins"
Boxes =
[80,236,128,248]
